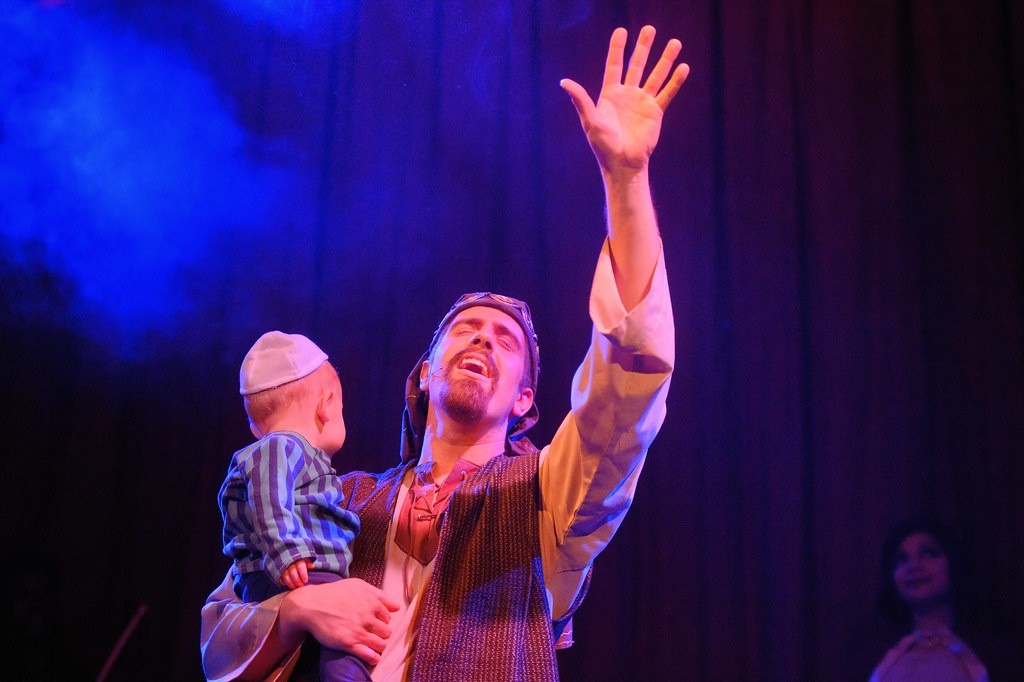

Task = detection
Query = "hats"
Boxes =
[400,292,541,464]
[239,330,328,396]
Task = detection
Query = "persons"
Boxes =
[833,517,1018,682]
[217,330,376,682]
[200,22,691,681]
